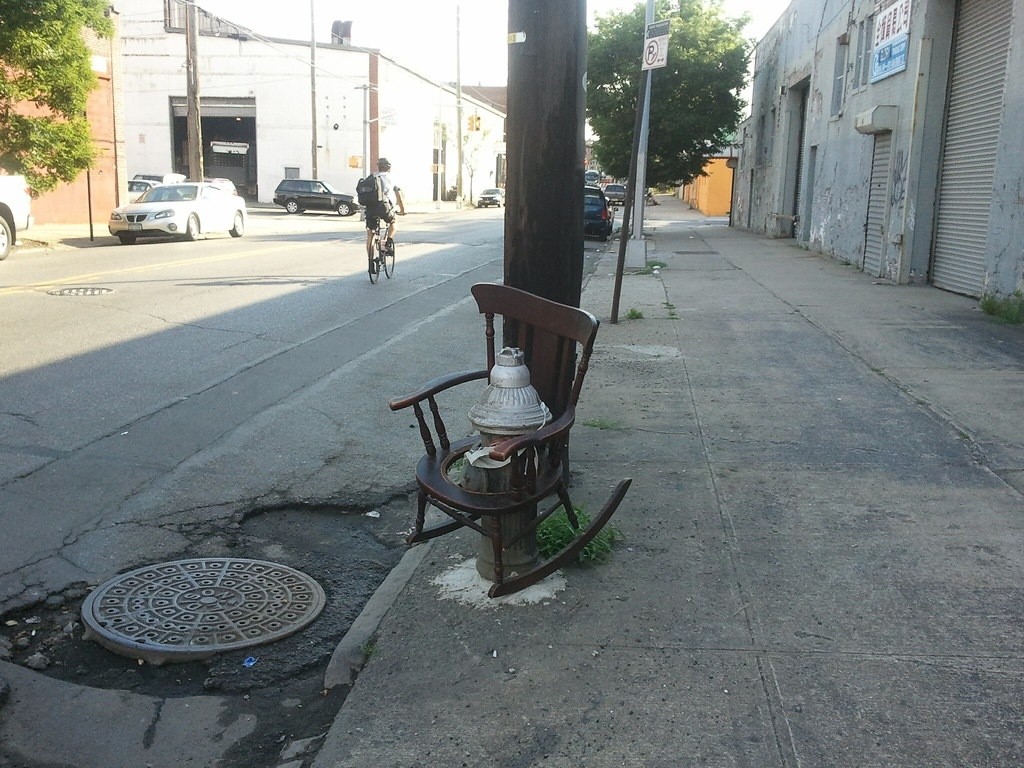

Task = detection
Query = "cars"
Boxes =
[124,173,238,200]
[109,182,247,245]
[587,179,598,187]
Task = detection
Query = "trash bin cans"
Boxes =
[644,194,653,206]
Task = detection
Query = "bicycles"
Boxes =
[365,210,407,284]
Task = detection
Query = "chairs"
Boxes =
[389,281,633,599]
[591,198,599,204]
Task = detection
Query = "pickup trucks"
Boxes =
[0,174,32,260]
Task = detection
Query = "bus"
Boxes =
[585,170,601,187]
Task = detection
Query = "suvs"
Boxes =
[478,188,505,207]
[273,179,360,216]
[603,184,626,207]
[584,186,612,241]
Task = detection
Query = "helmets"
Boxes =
[377,158,391,167]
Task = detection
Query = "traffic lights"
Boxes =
[468,114,481,131]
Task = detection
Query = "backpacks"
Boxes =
[356,173,383,205]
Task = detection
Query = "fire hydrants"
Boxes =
[457,346,555,581]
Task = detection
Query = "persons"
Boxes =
[366,158,406,274]
[312,185,322,193]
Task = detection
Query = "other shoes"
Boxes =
[385,238,394,256]
[368,262,375,272]
